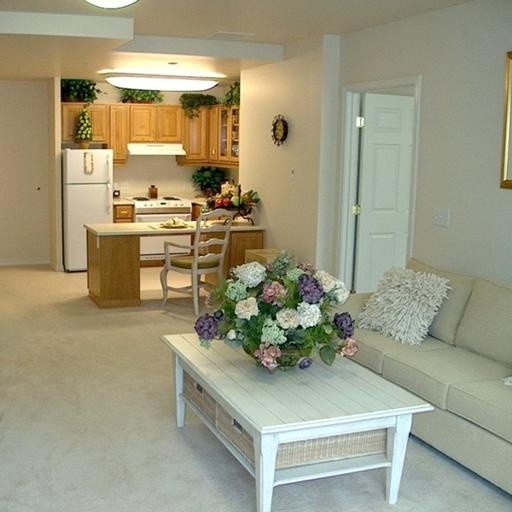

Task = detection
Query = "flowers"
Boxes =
[193,248,359,371]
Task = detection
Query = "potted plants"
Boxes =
[192,166,226,198]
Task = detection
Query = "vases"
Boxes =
[242,341,312,368]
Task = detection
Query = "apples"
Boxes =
[230,196,242,207]
[222,198,232,208]
[215,199,223,208]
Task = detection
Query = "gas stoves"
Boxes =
[124,195,192,208]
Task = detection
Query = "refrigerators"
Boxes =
[63,147,114,271]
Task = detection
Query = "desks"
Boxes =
[83,219,266,309]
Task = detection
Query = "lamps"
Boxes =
[103,76,223,92]
[84,0,139,9]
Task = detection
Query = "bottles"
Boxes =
[149,184,158,198]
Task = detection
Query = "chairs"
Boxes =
[160,207,234,323]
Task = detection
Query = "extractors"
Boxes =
[127,143,187,156]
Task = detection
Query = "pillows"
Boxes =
[356,267,453,348]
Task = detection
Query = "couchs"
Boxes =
[327,257,512,495]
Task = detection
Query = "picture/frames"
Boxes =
[500,52,512,189]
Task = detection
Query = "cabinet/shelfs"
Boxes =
[114,205,134,223]
[192,203,230,256]
[61,102,240,169]
